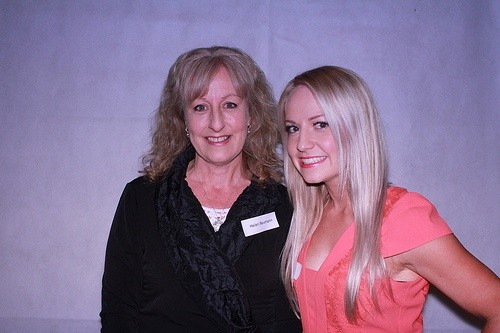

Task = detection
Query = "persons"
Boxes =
[276,65,500,333]
[96,45,304,333]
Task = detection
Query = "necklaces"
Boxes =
[193,165,245,221]
